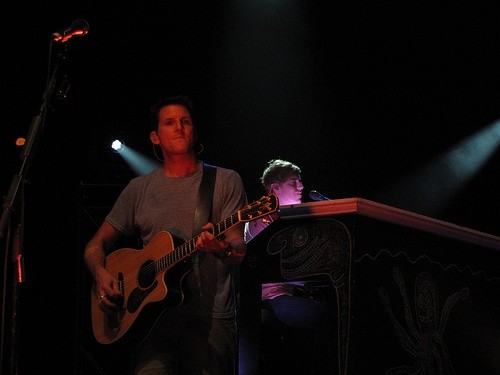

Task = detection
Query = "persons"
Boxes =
[84,97,248,375]
[239,159,319,375]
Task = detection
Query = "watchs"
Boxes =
[212,242,233,260]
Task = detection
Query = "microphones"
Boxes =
[309,190,330,200]
[52,18,89,42]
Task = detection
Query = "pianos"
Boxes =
[239,196,500,375]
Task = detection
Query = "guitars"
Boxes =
[90,194,279,354]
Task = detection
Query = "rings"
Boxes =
[100,295,106,300]
